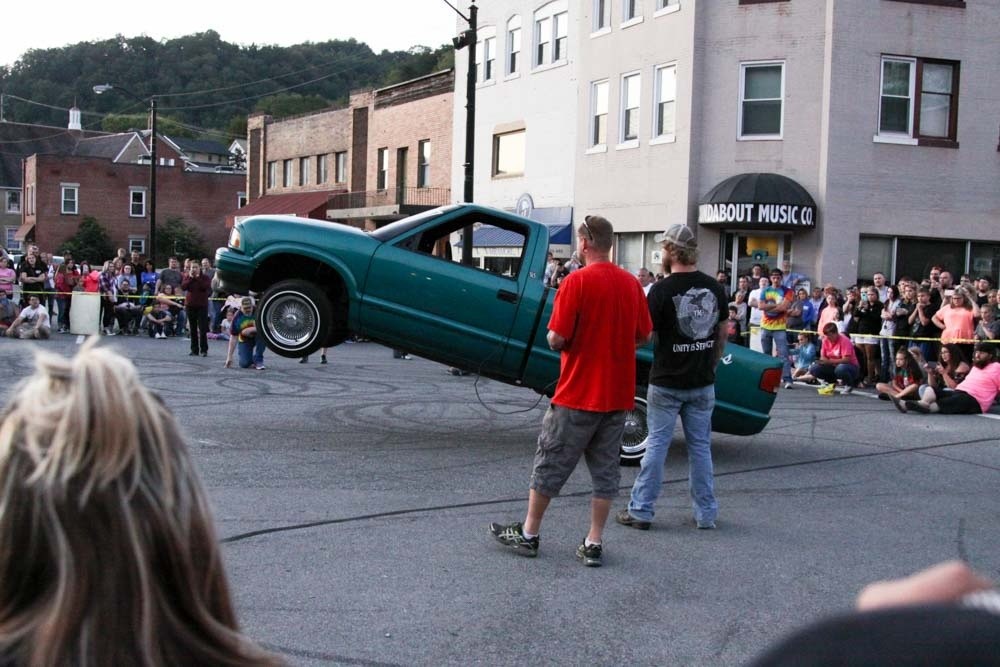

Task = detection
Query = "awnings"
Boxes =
[698,173,817,232]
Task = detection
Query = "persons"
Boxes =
[752,562,1000,667]
[182,259,213,358]
[0,244,256,341]
[542,251,583,289]
[0,331,292,667]
[446,367,469,376]
[224,298,266,370]
[346,334,370,343]
[718,265,1000,416]
[637,267,664,299]
[489,216,652,568]
[615,224,731,529]
[394,348,412,360]
[299,348,327,364]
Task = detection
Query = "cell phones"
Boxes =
[944,290,954,295]
[850,291,856,297]
[928,361,937,367]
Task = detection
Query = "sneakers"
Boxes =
[840,386,853,395]
[575,538,603,566]
[488,521,539,557]
[690,514,715,530]
[615,510,651,531]
[783,382,793,390]
[255,363,266,370]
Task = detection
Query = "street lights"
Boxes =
[138,154,160,269]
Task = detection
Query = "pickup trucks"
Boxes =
[209,201,786,469]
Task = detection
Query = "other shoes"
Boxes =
[299,356,308,364]
[905,400,931,414]
[103,327,186,338]
[188,351,198,356]
[877,393,890,400]
[890,395,907,413]
[320,355,328,364]
[201,352,207,358]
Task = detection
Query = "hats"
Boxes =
[771,269,783,276]
[242,298,252,306]
[976,342,996,353]
[654,224,696,249]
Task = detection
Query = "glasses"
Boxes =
[583,216,593,241]
[939,351,949,354]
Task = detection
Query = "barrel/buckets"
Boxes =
[69,290,101,335]
